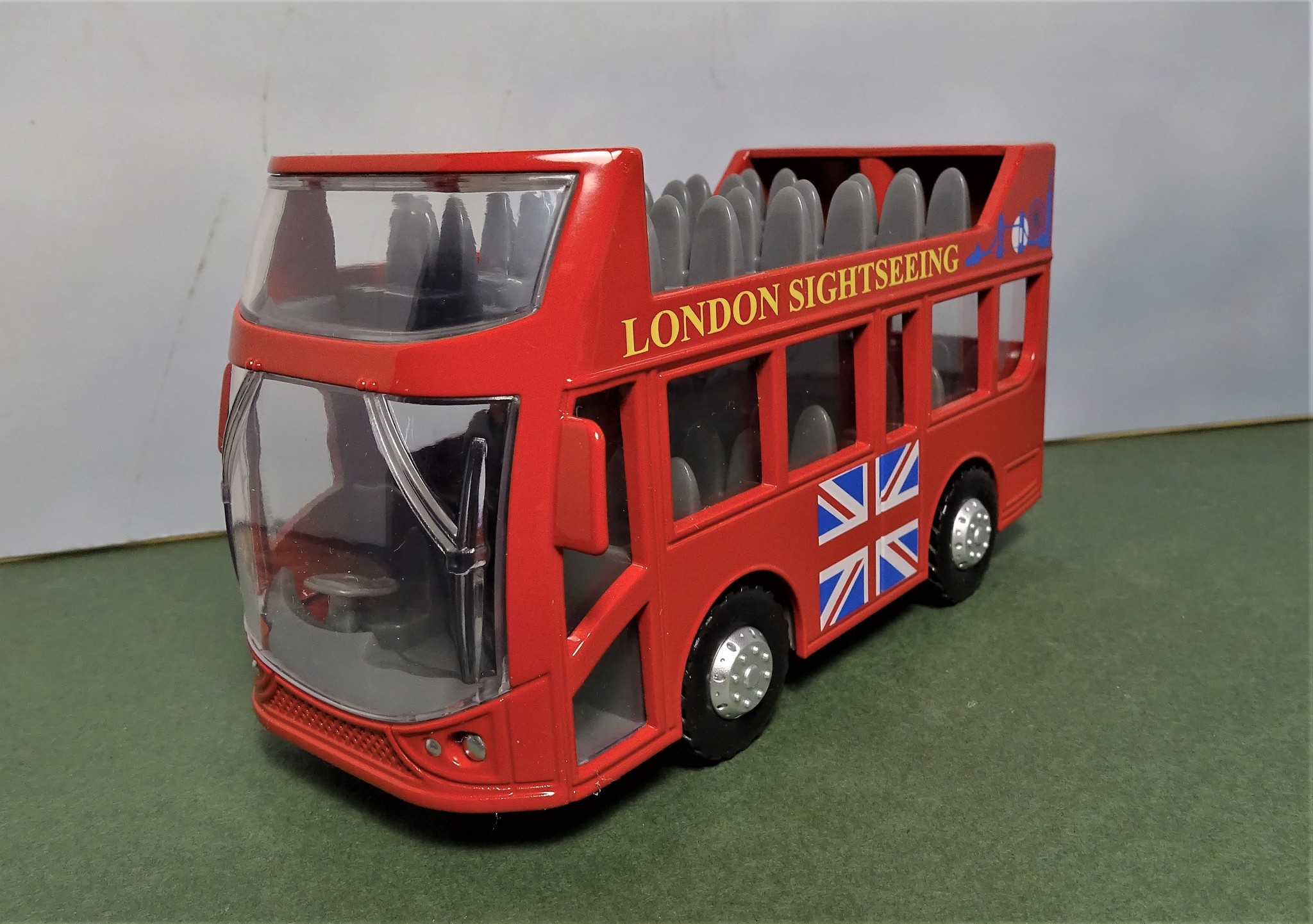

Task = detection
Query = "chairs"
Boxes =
[350,164,970,327]
[462,335,967,546]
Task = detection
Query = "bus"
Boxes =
[213,127,1059,814]
